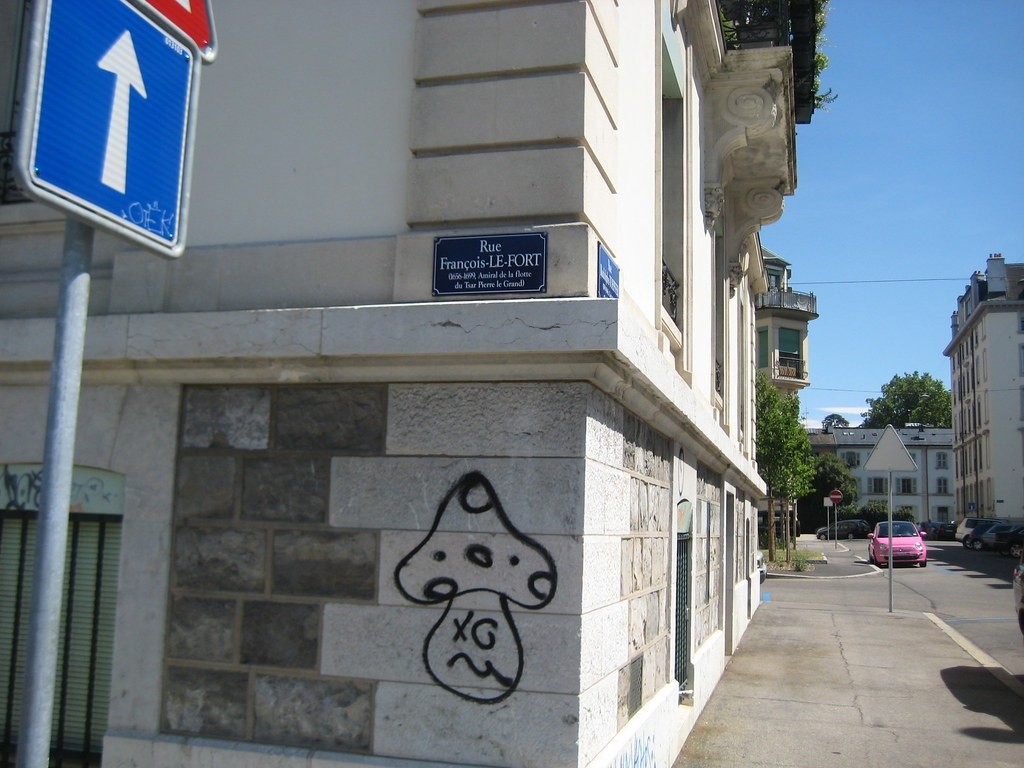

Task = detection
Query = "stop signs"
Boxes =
[830,490,843,504]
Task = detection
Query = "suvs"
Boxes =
[815,519,873,539]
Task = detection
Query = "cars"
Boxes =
[867,521,927,567]
[915,517,1024,560]
[1012,552,1024,639]
